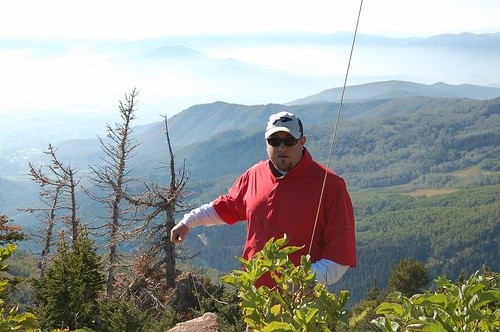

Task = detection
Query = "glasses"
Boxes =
[265,137,300,147]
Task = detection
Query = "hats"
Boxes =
[265,111,303,140]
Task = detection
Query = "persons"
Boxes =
[170,111,358,293]
